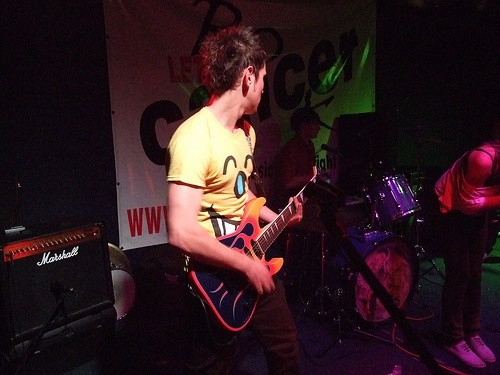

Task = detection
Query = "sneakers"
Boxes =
[467,335,496,363]
[445,343,487,368]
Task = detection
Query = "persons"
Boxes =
[276,107,322,278]
[433,139,500,369]
[168,25,303,375]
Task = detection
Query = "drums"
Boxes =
[362,174,422,224]
[342,225,419,323]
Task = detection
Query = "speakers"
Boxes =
[0,221,116,348]
[0,307,118,375]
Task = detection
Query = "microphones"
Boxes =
[320,144,340,155]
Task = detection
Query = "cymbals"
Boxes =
[108,243,137,320]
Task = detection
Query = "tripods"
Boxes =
[299,233,349,319]
[400,185,445,299]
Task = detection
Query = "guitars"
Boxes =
[187,165,319,332]
